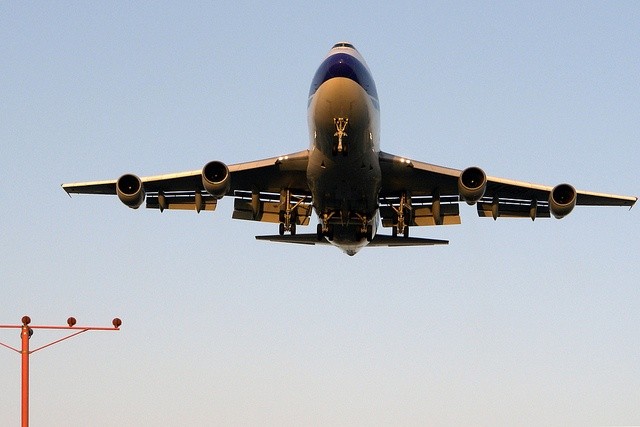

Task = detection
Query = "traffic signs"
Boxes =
[0,314,122,426]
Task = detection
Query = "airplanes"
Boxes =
[61,41,638,257]
